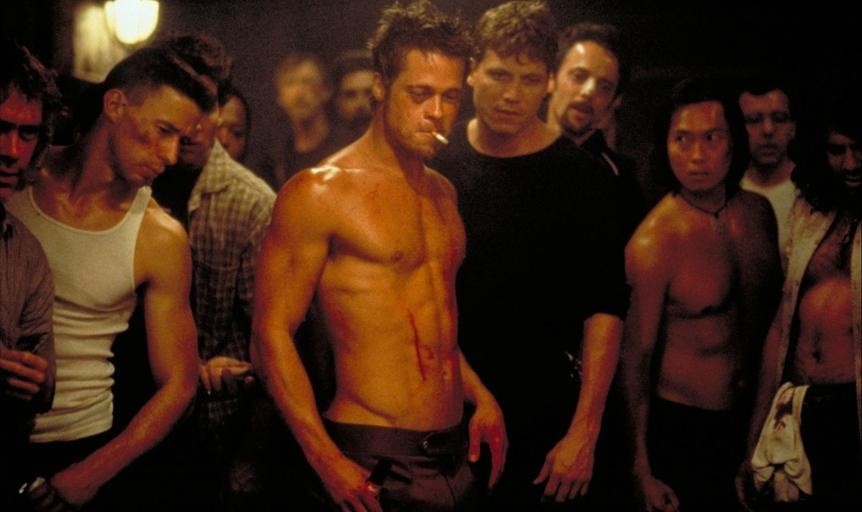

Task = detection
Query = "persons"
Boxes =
[249,0,633,510]
[618,75,862,511]
[3,31,275,510]
